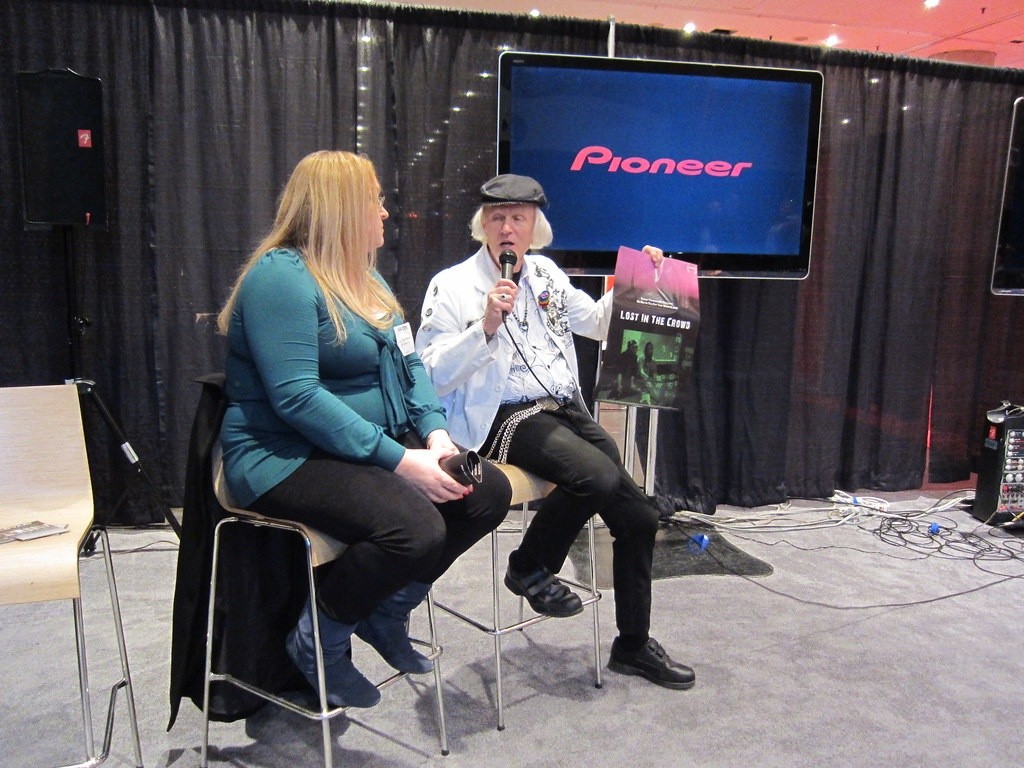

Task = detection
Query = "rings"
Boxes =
[501,294,505,299]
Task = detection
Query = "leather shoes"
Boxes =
[606,636,696,690]
[503,549,584,618]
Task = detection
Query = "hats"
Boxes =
[479,174,548,205]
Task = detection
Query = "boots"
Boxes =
[355,581,435,675]
[285,594,381,708]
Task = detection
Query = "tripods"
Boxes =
[60,227,180,555]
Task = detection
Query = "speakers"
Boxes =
[12,67,110,227]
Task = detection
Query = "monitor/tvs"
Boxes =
[497,52,825,278]
[991,97,1024,297]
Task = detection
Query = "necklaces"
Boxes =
[512,281,528,331]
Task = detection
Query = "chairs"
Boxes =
[0,385,142,768]
[402,461,604,731]
[167,375,448,768]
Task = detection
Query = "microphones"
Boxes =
[498,249,517,322]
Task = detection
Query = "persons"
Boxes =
[616,340,656,396]
[218,151,513,710]
[412,175,695,689]
